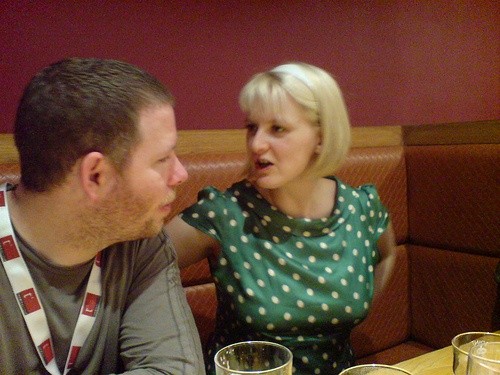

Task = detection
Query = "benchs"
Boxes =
[0,119,500,365]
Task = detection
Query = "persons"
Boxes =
[160,62,397,375]
[0,56,205,375]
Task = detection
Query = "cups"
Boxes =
[451,332,500,375]
[339,364,412,375]
[213,340,293,375]
[466,342,500,375]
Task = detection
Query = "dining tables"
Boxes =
[392,330,500,375]
